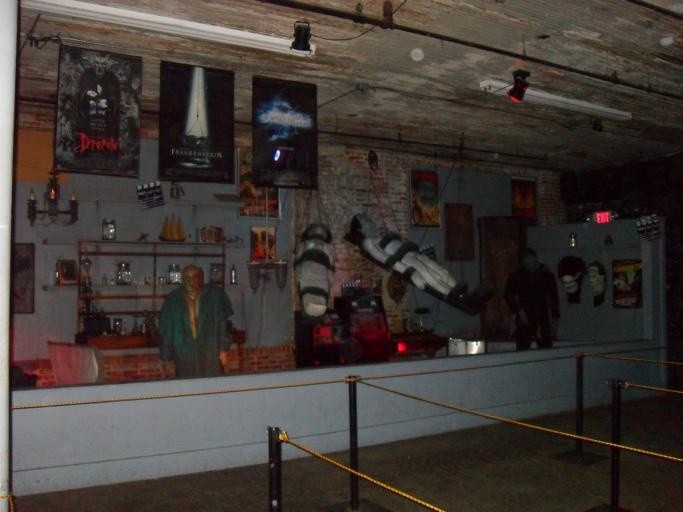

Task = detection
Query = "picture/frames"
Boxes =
[252,75,317,189]
[158,60,235,184]
[54,44,143,178]
[409,167,536,286]
[612,259,643,309]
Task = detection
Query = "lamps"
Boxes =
[28,185,78,226]
[246,259,290,294]
[289,20,312,56]
[507,31,530,106]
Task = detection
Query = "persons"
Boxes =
[504,245,559,354]
[349,213,491,316]
[157,263,233,379]
[291,219,336,317]
[629,260,642,293]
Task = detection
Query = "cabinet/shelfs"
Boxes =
[74,241,245,349]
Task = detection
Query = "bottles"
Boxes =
[102,261,223,286]
[102,220,117,240]
[162,211,184,240]
